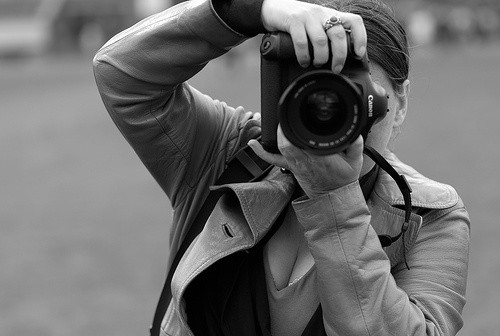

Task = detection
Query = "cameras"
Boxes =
[259,31,389,155]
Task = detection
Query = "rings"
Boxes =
[323,16,344,32]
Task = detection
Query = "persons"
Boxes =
[92,0,471,336]
